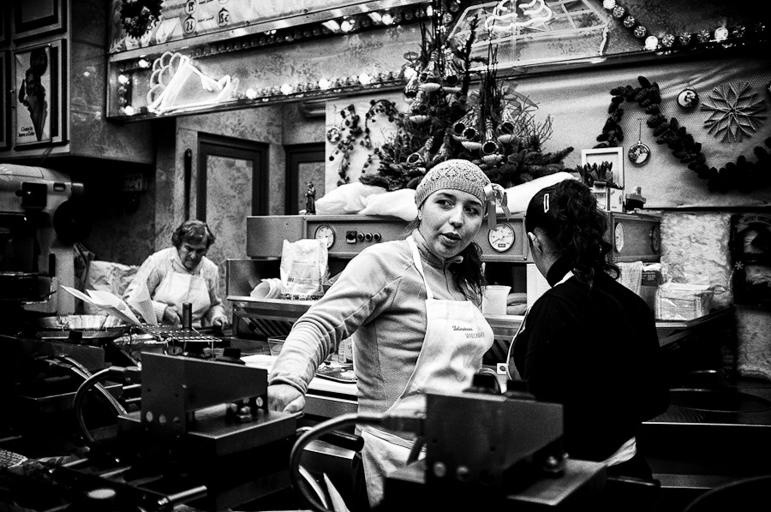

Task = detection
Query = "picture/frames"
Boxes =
[0,50,12,152]
[11,0,68,47]
[581,147,624,189]
[10,38,68,152]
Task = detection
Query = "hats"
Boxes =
[414,158,492,210]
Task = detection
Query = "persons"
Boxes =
[256,157,512,509]
[502,178,676,482]
[119,218,233,333]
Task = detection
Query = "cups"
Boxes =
[479,283,512,315]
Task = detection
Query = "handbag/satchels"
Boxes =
[279,237,329,300]
[654,282,713,322]
